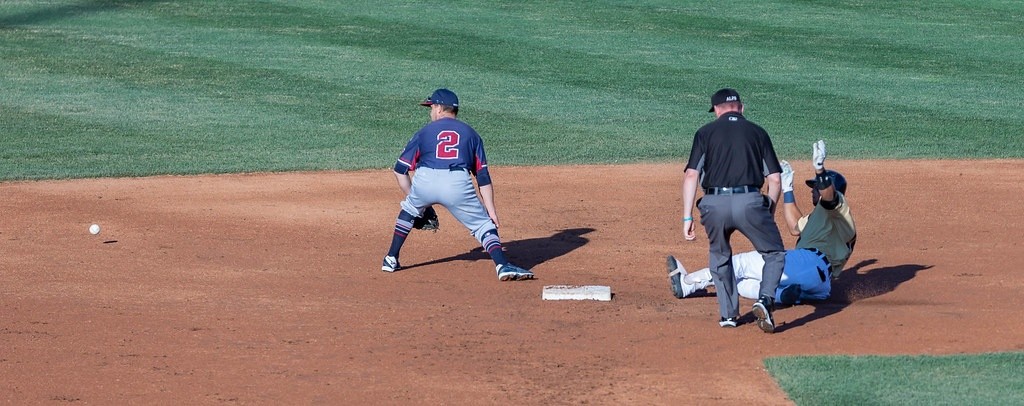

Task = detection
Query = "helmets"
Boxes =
[805,170,847,195]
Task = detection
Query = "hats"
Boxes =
[708,89,740,113]
[420,89,459,107]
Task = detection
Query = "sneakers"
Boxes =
[496,263,534,281]
[666,255,695,298]
[752,296,775,333]
[719,317,738,327]
[382,255,401,272]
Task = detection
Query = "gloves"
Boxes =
[812,140,827,170]
[779,160,795,193]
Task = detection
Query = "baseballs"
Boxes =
[89,225,100,235]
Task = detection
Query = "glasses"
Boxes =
[426,96,443,104]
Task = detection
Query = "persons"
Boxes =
[681,88,783,334]
[381,88,535,282]
[665,139,858,307]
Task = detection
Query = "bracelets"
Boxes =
[683,215,693,221]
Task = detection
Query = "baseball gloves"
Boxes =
[413,206,439,233]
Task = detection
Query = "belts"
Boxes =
[450,167,471,174]
[704,186,759,195]
[805,248,833,279]
